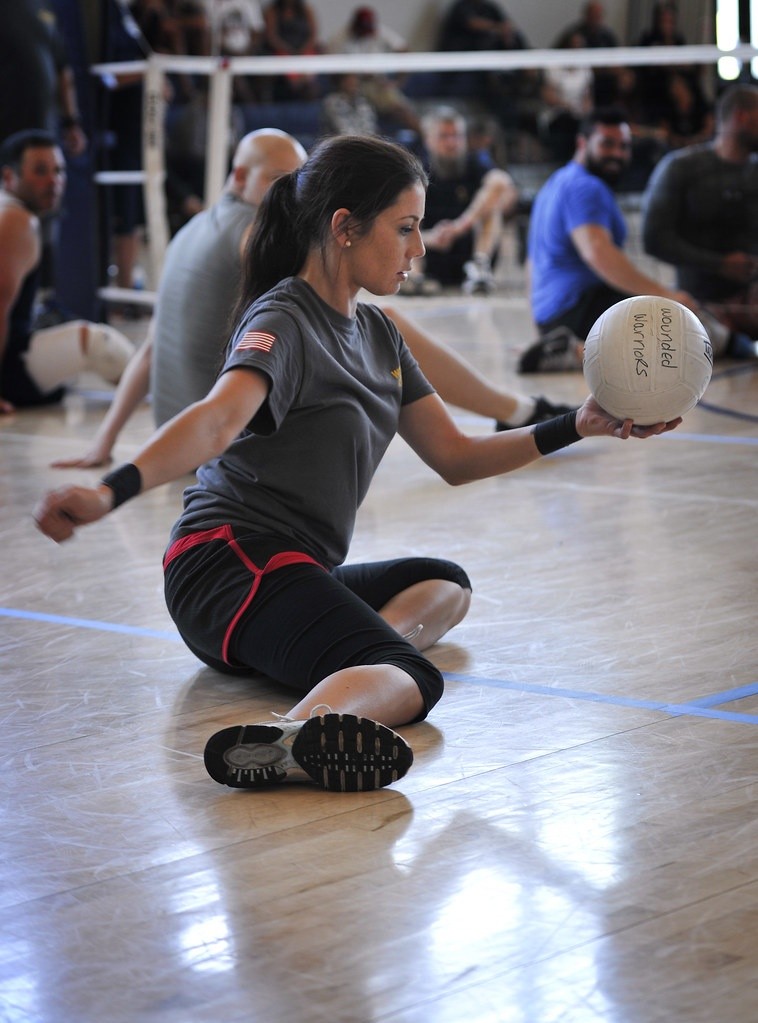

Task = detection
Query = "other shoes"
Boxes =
[712,329,758,369]
[401,273,426,295]
[516,326,574,375]
[461,262,495,295]
[203,704,414,792]
[494,396,584,433]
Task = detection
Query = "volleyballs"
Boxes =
[581,295,714,428]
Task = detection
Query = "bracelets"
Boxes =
[535,410,584,454]
[100,463,142,510]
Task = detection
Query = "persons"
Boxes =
[0,0,758,414]
[47,128,588,469]
[30,135,682,790]
